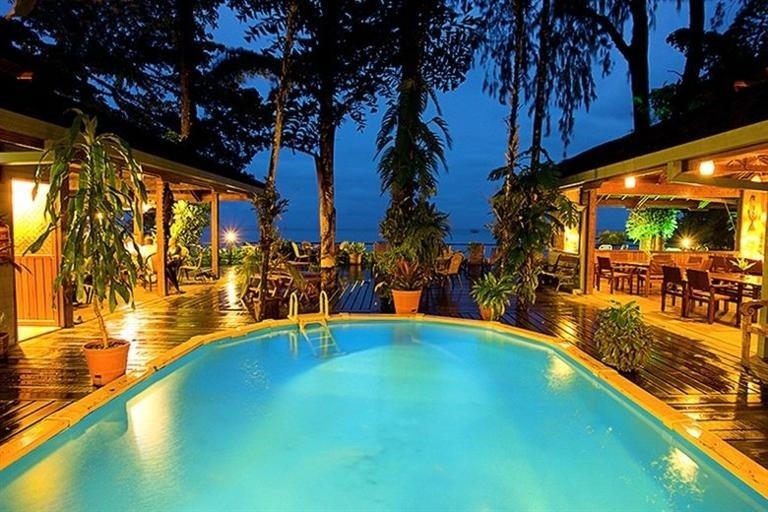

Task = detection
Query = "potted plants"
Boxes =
[466,271,520,323]
[382,256,435,314]
[0,311,13,356]
[19,105,154,392]
[340,241,365,265]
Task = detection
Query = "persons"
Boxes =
[125,234,183,286]
[470,244,503,265]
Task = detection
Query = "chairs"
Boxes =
[289,241,319,270]
[536,250,579,292]
[594,251,766,324]
[437,244,502,286]
[144,244,202,292]
